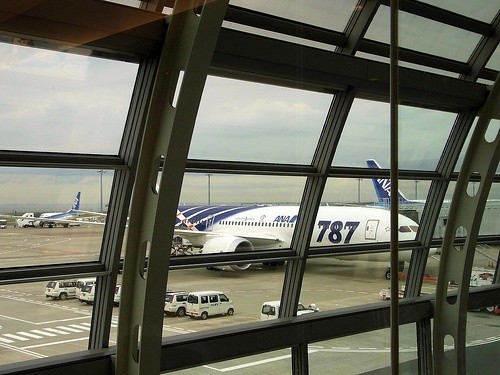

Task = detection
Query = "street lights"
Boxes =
[356,178,363,204]
[97,169,107,213]
[471,183,476,196]
[413,181,420,200]
[205,173,212,206]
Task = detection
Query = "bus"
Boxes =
[0,220,7,229]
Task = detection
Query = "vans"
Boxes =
[75,279,96,299]
[44,279,78,300]
[185,290,235,320]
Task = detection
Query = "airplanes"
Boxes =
[172,204,438,279]
[365,159,500,238]
[12,191,81,228]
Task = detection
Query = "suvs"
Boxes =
[164,292,189,317]
[79,284,122,306]
[261,300,316,320]
